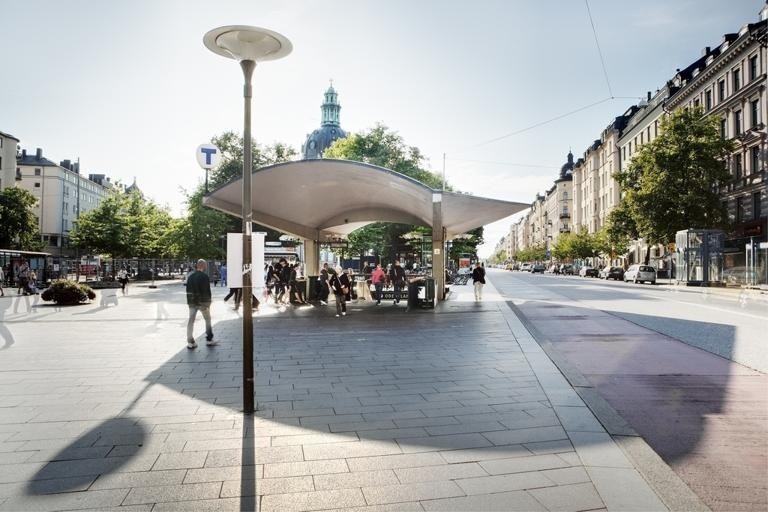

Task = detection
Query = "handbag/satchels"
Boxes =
[342,287,348,294]
[369,284,377,292]
[395,280,405,288]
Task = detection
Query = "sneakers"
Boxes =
[320,300,328,306]
[206,340,214,346]
[376,299,398,306]
[186,342,197,349]
[335,314,339,317]
[341,312,345,316]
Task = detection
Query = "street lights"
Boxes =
[201,22,297,416]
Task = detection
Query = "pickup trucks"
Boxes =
[528,265,546,274]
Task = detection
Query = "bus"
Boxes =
[0,249,53,285]
[79,254,102,275]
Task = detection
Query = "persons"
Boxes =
[473,261,486,303]
[224,257,407,318]
[182,257,222,350]
[0,259,38,296]
[117,264,129,291]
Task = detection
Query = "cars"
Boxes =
[520,263,530,272]
[599,265,624,280]
[485,261,518,270]
[622,264,657,285]
[547,261,576,277]
[577,266,597,278]
